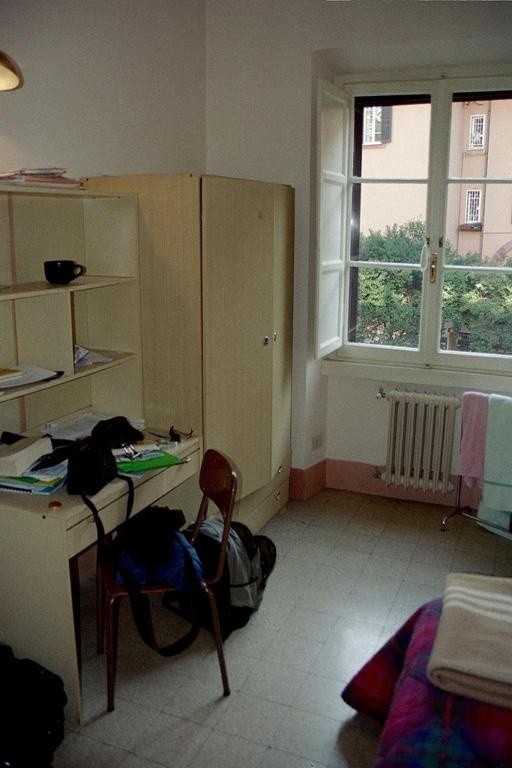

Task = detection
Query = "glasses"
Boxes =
[169,426,193,443]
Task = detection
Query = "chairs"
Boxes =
[94,448,240,712]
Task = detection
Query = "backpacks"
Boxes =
[161,518,276,644]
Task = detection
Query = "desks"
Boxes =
[0,428,202,729]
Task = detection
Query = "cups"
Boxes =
[44,260,85,286]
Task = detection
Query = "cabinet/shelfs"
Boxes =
[82,172,296,533]
[1,183,146,477]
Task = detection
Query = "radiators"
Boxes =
[372,386,461,498]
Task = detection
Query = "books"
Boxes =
[111,437,190,478]
[0,430,68,496]
[0,163,87,190]
[0,362,65,397]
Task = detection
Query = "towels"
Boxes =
[459,390,512,530]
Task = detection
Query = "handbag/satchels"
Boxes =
[109,505,203,657]
[30,434,134,566]
